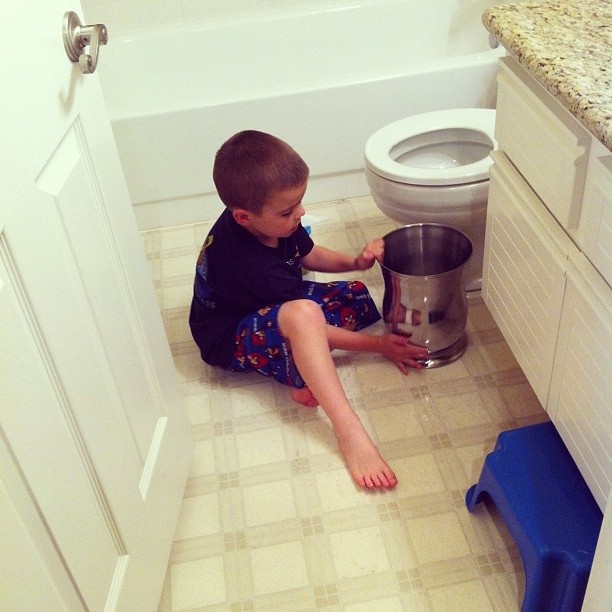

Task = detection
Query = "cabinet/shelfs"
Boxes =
[478,57,612,513]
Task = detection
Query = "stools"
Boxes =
[464,420,605,612]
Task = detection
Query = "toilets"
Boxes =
[363,109,497,292]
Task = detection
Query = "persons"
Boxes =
[188,130,431,490]
